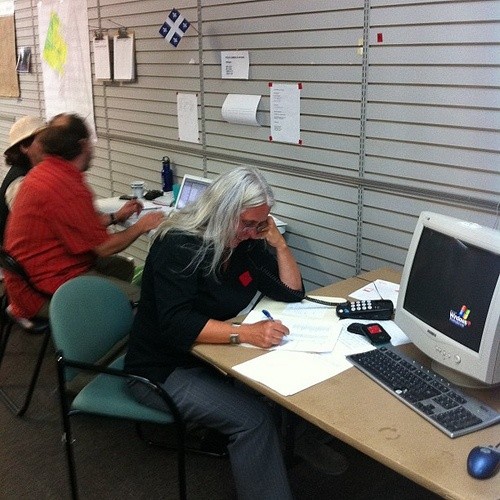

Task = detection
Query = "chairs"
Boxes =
[0,249,141,418]
[49,274,230,500]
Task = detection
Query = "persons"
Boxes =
[2,111,167,399]
[0,115,48,268]
[124,166,305,500]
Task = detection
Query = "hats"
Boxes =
[2,114,49,156]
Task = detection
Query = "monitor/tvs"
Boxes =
[175,173,213,212]
[395,212,500,388]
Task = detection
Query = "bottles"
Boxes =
[162,156,173,192]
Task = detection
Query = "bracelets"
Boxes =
[229,321,242,344]
[110,212,117,226]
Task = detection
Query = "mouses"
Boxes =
[467,442,500,479]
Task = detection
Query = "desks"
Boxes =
[176,266,499,500]
[93,196,286,245]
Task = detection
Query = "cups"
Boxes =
[131,181,144,199]
[173,184,180,203]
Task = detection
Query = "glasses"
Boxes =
[239,221,270,234]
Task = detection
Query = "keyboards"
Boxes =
[348,346,499,438]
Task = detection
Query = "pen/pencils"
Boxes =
[143,206,162,210]
[262,309,287,337]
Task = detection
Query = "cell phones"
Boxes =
[348,322,366,335]
[361,322,391,344]
[119,195,137,200]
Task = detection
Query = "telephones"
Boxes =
[239,239,256,253]
